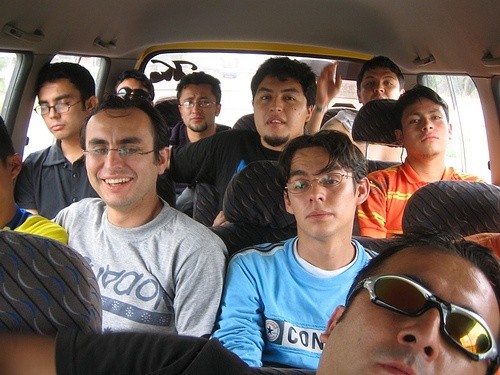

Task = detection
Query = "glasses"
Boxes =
[178,101,216,109]
[117,89,152,100]
[345,273,500,375]
[284,173,355,194]
[34,100,81,115]
[81,144,156,160]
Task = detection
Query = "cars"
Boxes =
[223,67,237,79]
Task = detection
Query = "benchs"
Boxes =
[208,160,500,253]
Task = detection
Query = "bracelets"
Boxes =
[314,105,324,113]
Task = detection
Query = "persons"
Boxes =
[211,129,381,369]
[50,95,228,337]
[0,55,500,375]
